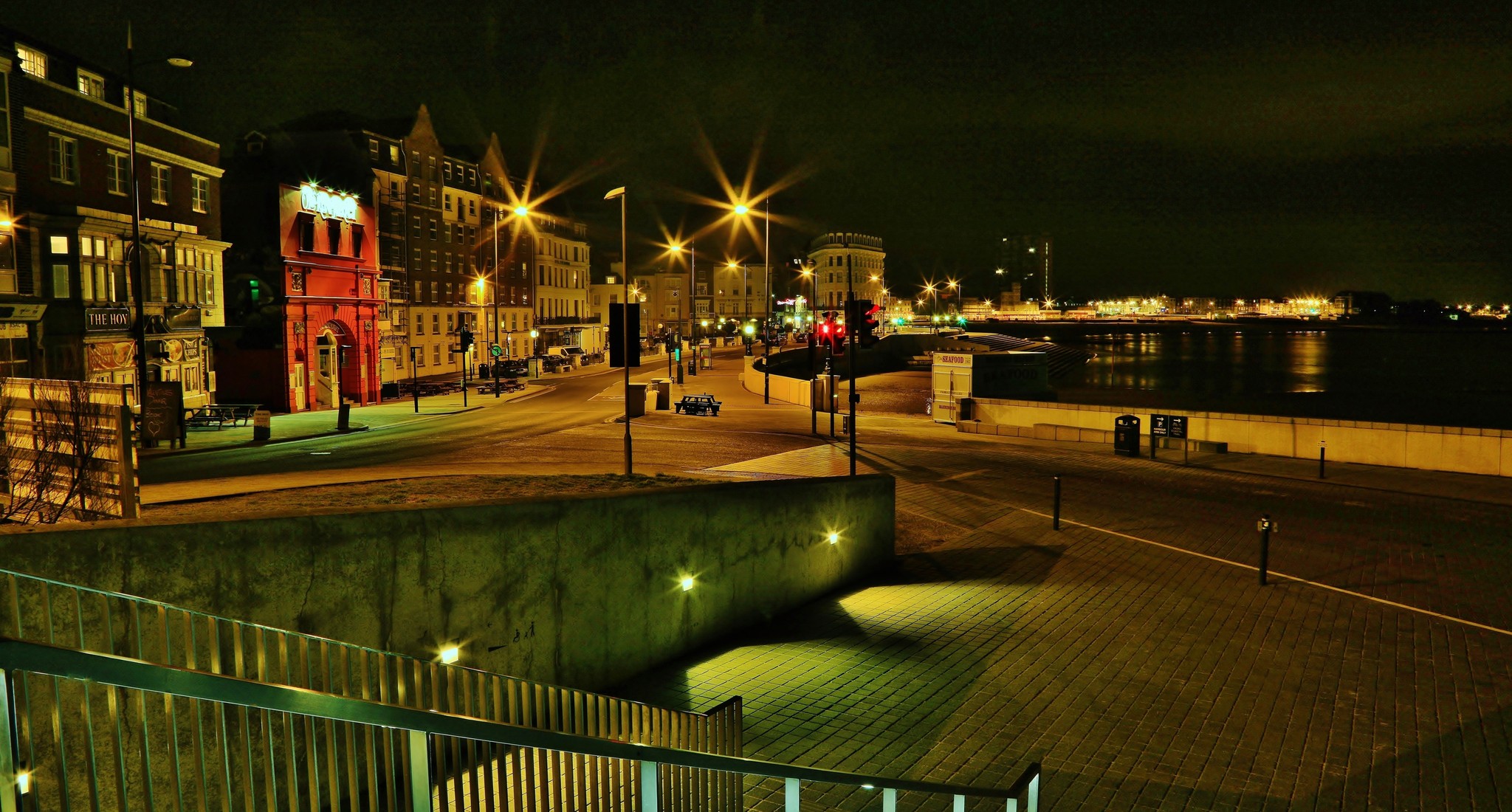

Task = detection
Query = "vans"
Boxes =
[548,345,588,367]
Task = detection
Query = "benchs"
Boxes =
[727,342,737,346]
[475,382,526,395]
[417,388,436,397]
[555,364,572,373]
[454,384,462,393]
[673,400,722,417]
[441,387,451,396]
[755,340,762,344]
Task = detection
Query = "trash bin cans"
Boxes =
[479,363,487,379]
[688,361,694,375]
[1114,414,1141,457]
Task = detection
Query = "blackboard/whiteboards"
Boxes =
[141,382,187,440]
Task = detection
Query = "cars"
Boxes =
[530,354,560,373]
[764,335,788,347]
[490,359,528,379]
[516,357,547,378]
[795,333,809,343]
[548,354,572,369]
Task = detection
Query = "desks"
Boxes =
[399,380,455,389]
[203,403,264,426]
[676,393,717,417]
[483,379,518,385]
[184,406,239,430]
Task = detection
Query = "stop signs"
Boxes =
[506,334,512,344]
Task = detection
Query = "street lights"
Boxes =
[337,344,352,428]
[531,325,542,379]
[593,325,596,354]
[951,282,961,315]
[729,263,750,357]
[409,345,422,414]
[927,286,933,334]
[882,300,887,336]
[805,257,819,435]
[671,244,697,373]
[125,47,197,449]
[604,182,633,474]
[735,201,771,406]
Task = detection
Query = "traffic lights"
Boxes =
[816,309,831,348]
[461,329,474,350]
[858,296,882,350]
[829,321,845,356]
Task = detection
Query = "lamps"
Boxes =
[199,336,210,346]
